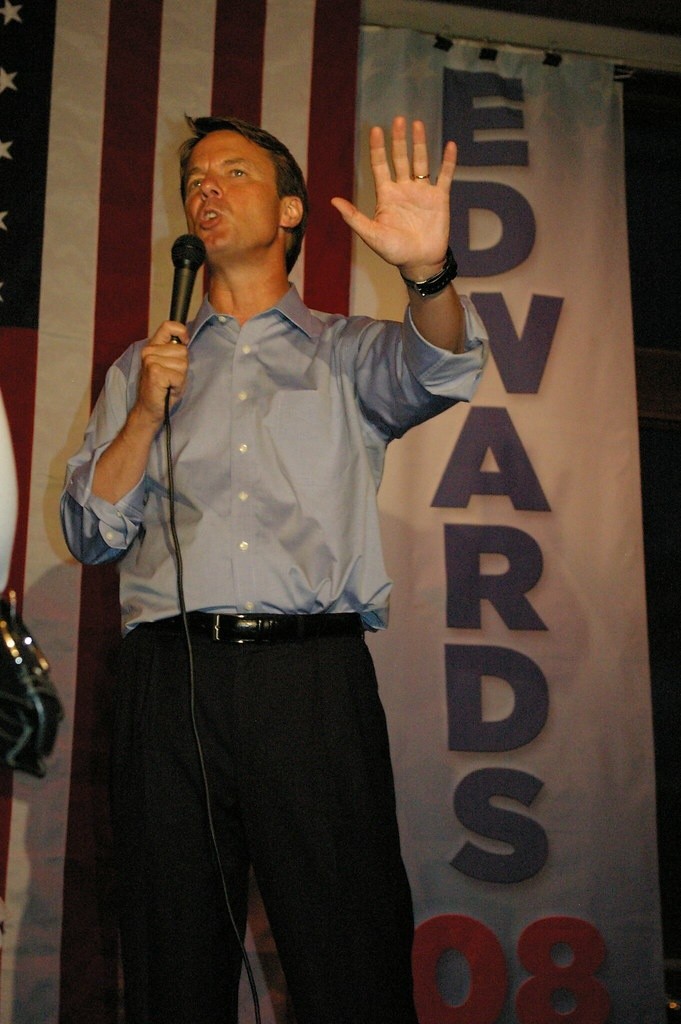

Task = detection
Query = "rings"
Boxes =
[412,175,429,179]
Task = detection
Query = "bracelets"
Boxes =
[401,246,459,298]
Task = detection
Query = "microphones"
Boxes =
[166,234,206,390]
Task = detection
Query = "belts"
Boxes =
[138,608,363,645]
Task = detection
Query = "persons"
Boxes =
[61,113,488,1023]
